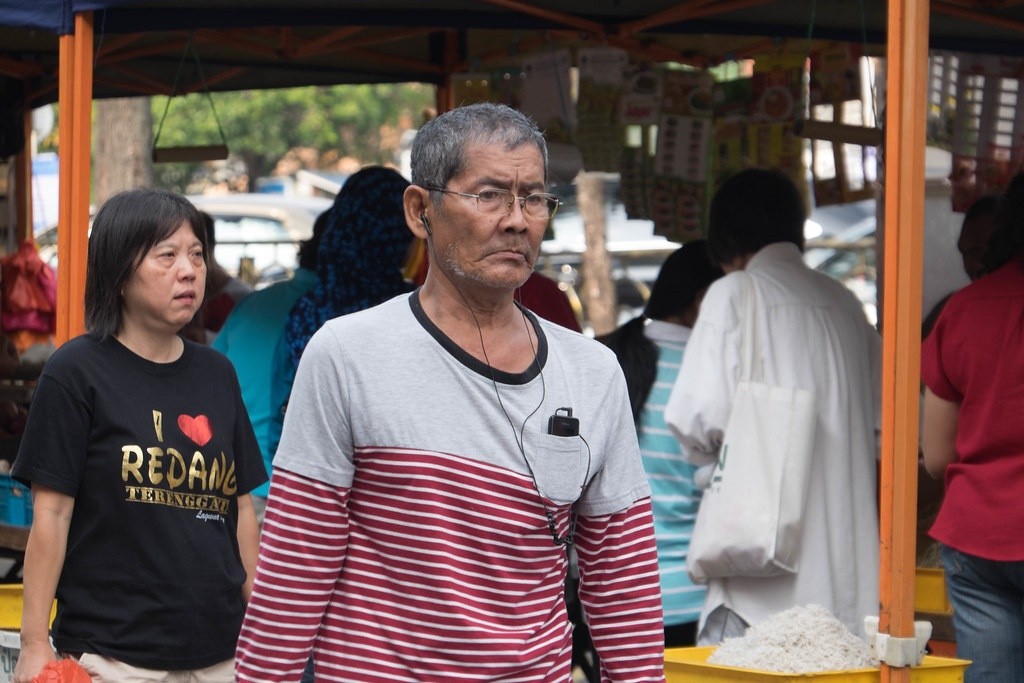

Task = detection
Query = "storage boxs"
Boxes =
[664,645,973,683]
[0,584,58,630]
[0,473,33,528]
[915,567,953,615]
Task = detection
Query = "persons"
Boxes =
[228,102,668,683]
[189,165,430,527]
[661,166,881,651]
[506,243,728,651]
[7,188,270,683]
[915,184,1024,683]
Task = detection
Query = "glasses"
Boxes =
[420,185,563,220]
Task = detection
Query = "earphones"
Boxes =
[421,212,432,236]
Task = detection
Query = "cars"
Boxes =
[32,146,975,342]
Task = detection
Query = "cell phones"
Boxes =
[547,416,579,438]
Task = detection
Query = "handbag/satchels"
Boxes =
[687,386,819,577]
